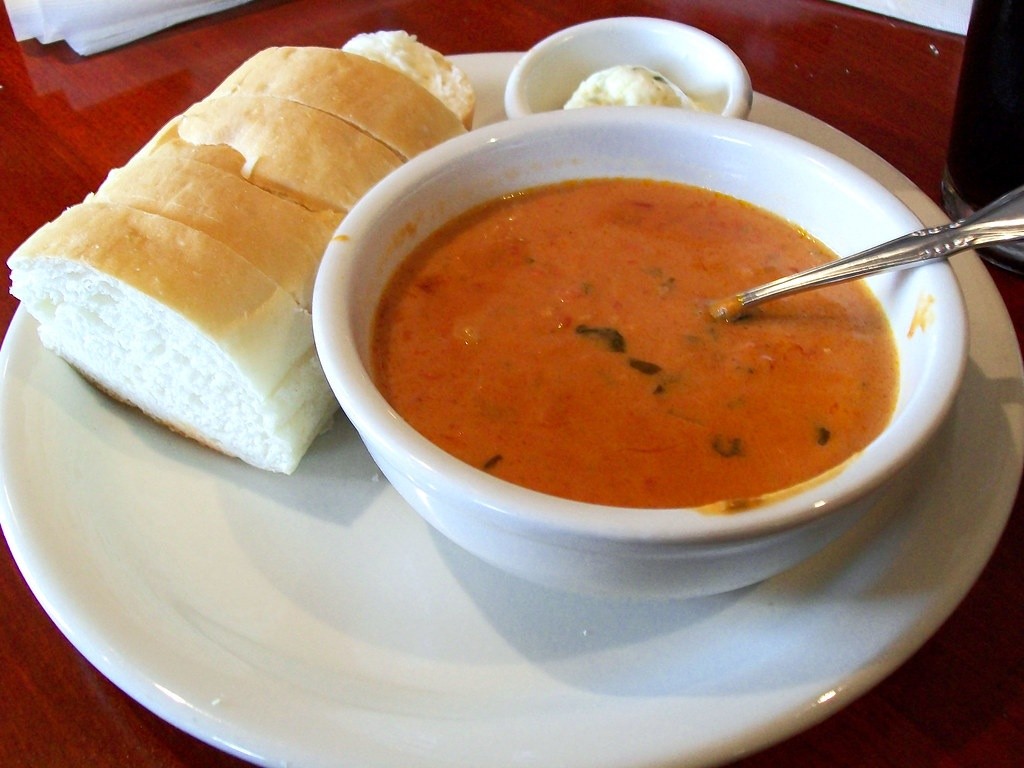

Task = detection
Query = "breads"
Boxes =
[6,30,498,476]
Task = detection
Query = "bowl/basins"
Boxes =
[504,16,753,122]
[312,105,970,600]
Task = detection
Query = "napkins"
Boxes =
[5,1,248,56]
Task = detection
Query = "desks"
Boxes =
[1,0,1023,766]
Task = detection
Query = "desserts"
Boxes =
[562,66,700,114]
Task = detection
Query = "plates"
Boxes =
[1,52,1024,768]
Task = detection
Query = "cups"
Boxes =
[935,1,1024,279]
